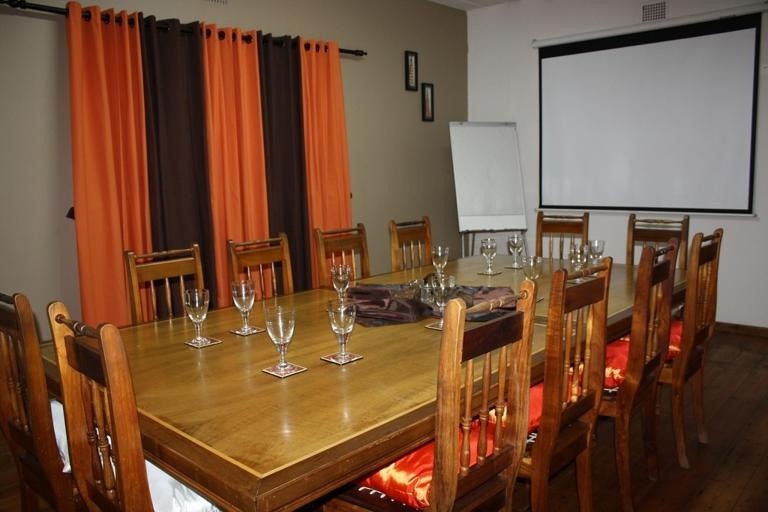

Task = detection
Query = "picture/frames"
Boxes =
[403,48,420,92]
[421,81,436,122]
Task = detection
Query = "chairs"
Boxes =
[534,212,590,259]
[46,300,221,512]
[486,257,614,512]
[619,226,726,476]
[387,216,433,272]
[316,278,539,511]
[123,243,205,326]
[313,222,372,288]
[624,212,692,272]
[226,231,294,305]
[596,237,682,512]
[1,290,113,512]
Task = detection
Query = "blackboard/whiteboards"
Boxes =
[448,121,528,233]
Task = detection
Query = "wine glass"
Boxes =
[481,234,545,303]
[404,244,456,329]
[568,241,603,289]
[185,289,209,347]
[328,262,352,304]
[328,296,356,362]
[263,303,296,374]
[231,276,257,334]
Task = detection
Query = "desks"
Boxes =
[21,252,691,512]
